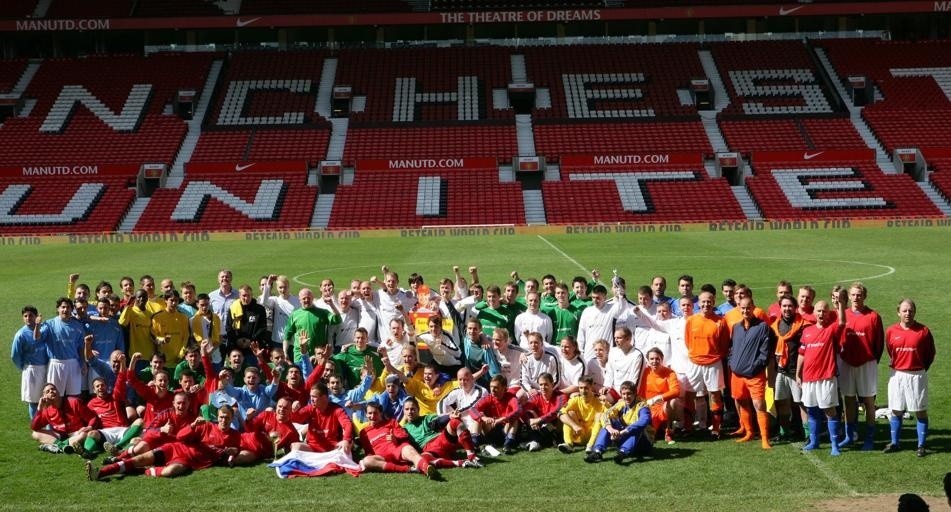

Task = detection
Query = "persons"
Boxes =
[260,273,300,346]
[33,297,88,408]
[381,353,490,417]
[66,273,113,306]
[452,264,515,343]
[314,279,341,315]
[606,275,635,312]
[101,392,196,466]
[468,375,524,456]
[519,332,561,393]
[398,397,486,469]
[281,288,342,365]
[768,280,793,323]
[71,299,91,330]
[586,340,610,387]
[569,276,595,319]
[651,275,675,315]
[177,281,198,318]
[770,294,813,443]
[256,276,274,334]
[84,334,136,403]
[207,270,239,336]
[440,291,500,387]
[638,347,681,445]
[119,276,137,310]
[334,327,382,385]
[218,367,281,422]
[696,283,722,315]
[290,384,353,459]
[88,297,125,395]
[558,375,612,454]
[684,292,730,438]
[574,269,620,366]
[235,397,299,466]
[725,284,767,437]
[173,346,205,384]
[328,289,358,352]
[475,280,527,322]
[617,285,652,354]
[326,355,375,418]
[74,284,91,299]
[188,293,222,368]
[599,326,642,403]
[174,371,201,413]
[344,374,407,423]
[139,274,164,316]
[224,284,267,354]
[11,305,48,421]
[829,285,858,442]
[795,291,847,457]
[31,383,97,454]
[882,298,937,457]
[194,339,236,410]
[583,381,654,464]
[516,278,539,311]
[667,275,700,317]
[542,283,582,343]
[416,316,463,380]
[727,298,773,449]
[117,288,155,374]
[358,366,388,402]
[795,285,816,325]
[452,267,478,309]
[149,289,190,367]
[158,279,178,300]
[396,346,442,396]
[348,281,377,342]
[520,373,569,451]
[85,405,241,482]
[349,278,361,291]
[72,354,144,458]
[480,326,524,395]
[321,360,336,379]
[377,303,416,373]
[523,329,586,395]
[347,272,417,343]
[435,277,465,347]
[382,264,439,299]
[248,340,332,408]
[126,352,174,430]
[633,296,697,395]
[644,301,673,366]
[360,402,437,480]
[510,269,600,316]
[259,347,286,384]
[718,278,736,315]
[838,283,885,450]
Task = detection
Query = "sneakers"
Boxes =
[83,461,100,482]
[468,457,487,468]
[614,451,628,464]
[675,428,692,440]
[102,455,119,465]
[72,440,91,460]
[502,445,512,455]
[38,444,58,453]
[711,431,720,441]
[768,434,791,446]
[408,465,422,474]
[528,441,543,452]
[916,447,926,457]
[63,445,75,455]
[839,435,853,447]
[861,436,875,451]
[425,464,441,480]
[585,443,592,453]
[480,444,495,457]
[583,450,604,463]
[103,442,119,457]
[664,436,676,446]
[799,438,821,451]
[462,458,476,469]
[884,444,900,454]
[557,442,575,455]
[829,445,841,457]
